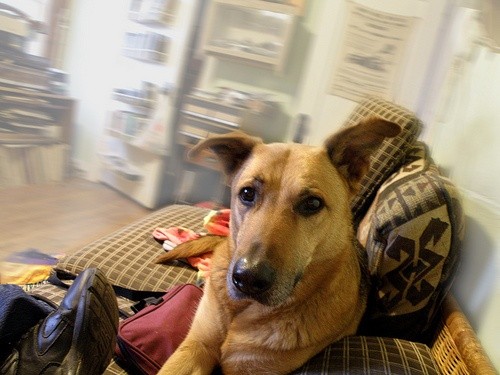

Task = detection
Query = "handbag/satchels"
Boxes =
[47,268,203,375]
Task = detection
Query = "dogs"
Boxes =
[155,117,402,375]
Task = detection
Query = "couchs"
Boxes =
[0,201,500,374]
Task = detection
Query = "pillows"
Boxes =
[352,152,465,339]
[335,90,426,233]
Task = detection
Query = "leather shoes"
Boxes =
[0,268,119,374]
